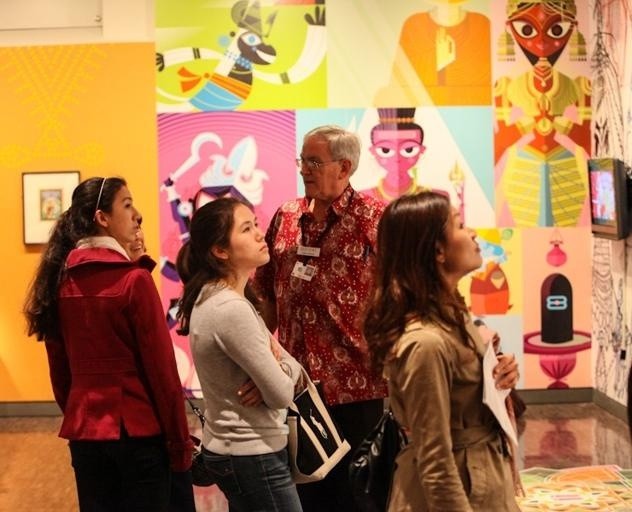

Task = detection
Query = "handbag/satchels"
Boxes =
[287,380,352,484]
[348,412,399,512]
[188,435,216,488]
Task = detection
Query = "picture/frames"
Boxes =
[22,171,80,245]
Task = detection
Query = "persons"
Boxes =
[362,192,527,512]
[124,218,197,510]
[242,129,391,511]
[23,176,198,512]
[175,197,307,512]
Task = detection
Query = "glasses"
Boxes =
[296,158,336,169]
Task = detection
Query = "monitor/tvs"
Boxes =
[587,157,632,240]
